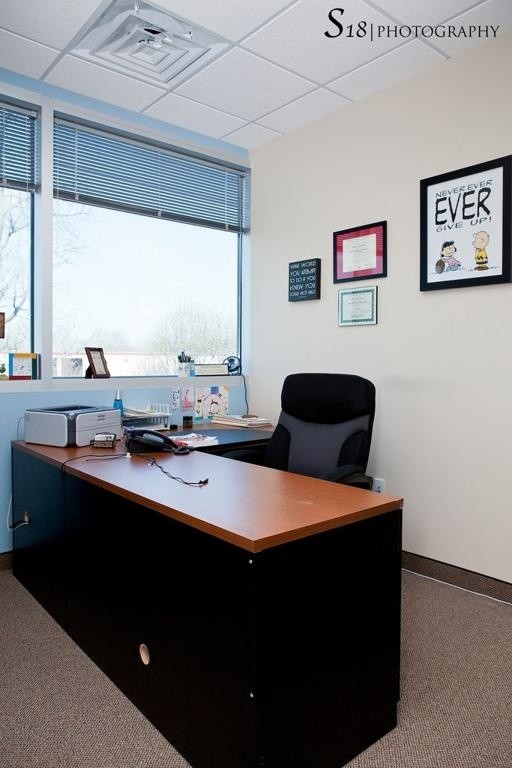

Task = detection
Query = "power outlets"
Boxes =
[372,478,385,494]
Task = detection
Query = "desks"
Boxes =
[10,417,403,766]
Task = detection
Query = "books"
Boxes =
[168,432,218,448]
[211,413,272,427]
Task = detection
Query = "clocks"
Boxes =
[8,352,41,381]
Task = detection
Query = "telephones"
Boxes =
[122,429,194,454]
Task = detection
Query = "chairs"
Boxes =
[225,372,377,489]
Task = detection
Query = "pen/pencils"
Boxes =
[177,351,191,364]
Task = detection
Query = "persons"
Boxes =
[437,240,460,276]
[472,232,491,271]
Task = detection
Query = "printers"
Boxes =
[24,404,122,448]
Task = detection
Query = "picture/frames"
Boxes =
[418,153,512,292]
[335,286,379,329]
[332,218,387,286]
[193,385,230,420]
[85,346,111,379]
